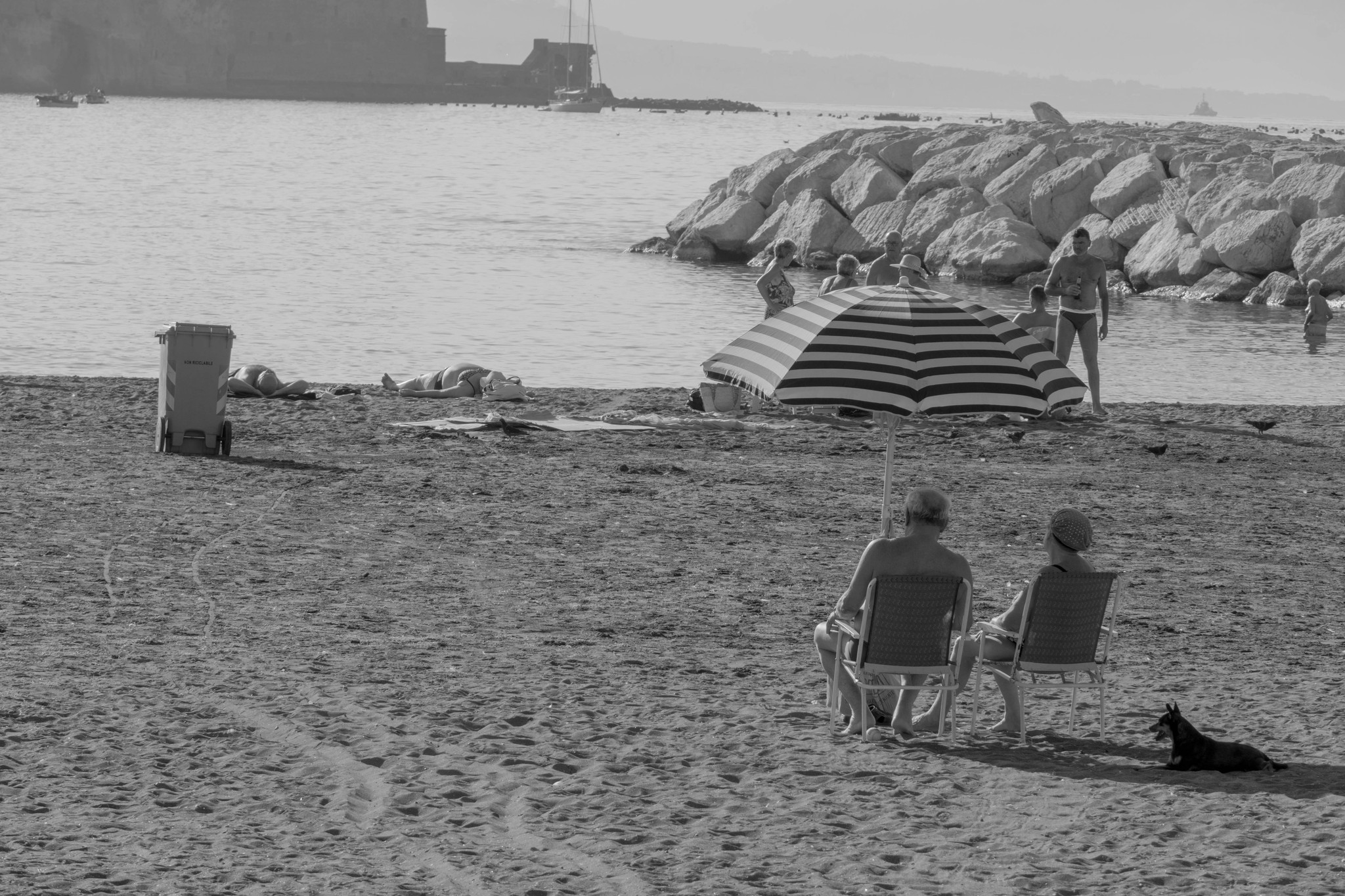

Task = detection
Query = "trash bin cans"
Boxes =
[153,322,238,455]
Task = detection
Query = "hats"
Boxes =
[1049,507,1094,552]
[889,254,929,279]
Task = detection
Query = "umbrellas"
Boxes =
[699,275,1092,538]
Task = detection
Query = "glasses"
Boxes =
[884,240,901,246]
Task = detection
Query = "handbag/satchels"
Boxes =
[860,671,903,714]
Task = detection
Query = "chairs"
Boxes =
[830,575,972,742]
[970,571,1121,745]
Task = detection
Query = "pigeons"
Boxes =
[1140,443,1169,460]
[1243,419,1277,436]
[998,427,1027,447]
[497,419,529,439]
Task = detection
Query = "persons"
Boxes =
[890,254,931,290]
[227,364,309,399]
[1011,284,1058,330]
[1044,226,1109,415]
[865,230,904,286]
[1303,279,1333,325]
[911,508,1097,737]
[381,363,535,398]
[756,238,798,321]
[813,489,974,737]
[817,253,859,297]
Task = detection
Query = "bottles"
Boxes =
[1074,276,1081,300]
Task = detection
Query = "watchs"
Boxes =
[833,606,838,618]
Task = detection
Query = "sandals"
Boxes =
[840,703,893,726]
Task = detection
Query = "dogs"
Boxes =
[1129,700,1289,775]
